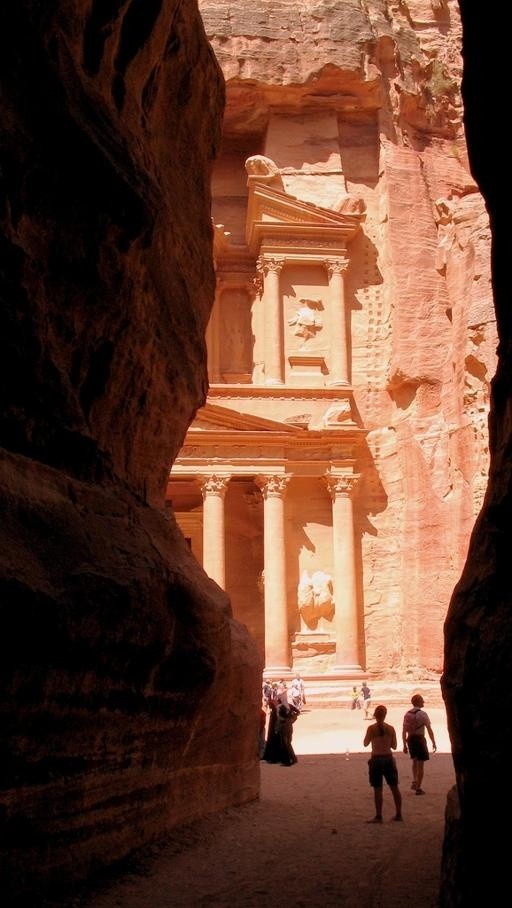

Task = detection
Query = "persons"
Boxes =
[275,703,300,765]
[359,681,374,719]
[263,698,289,763]
[402,693,437,796]
[263,670,307,714]
[351,686,361,709]
[364,704,404,823]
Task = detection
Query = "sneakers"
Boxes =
[367,814,383,823]
[391,813,402,821]
[410,783,426,795]
